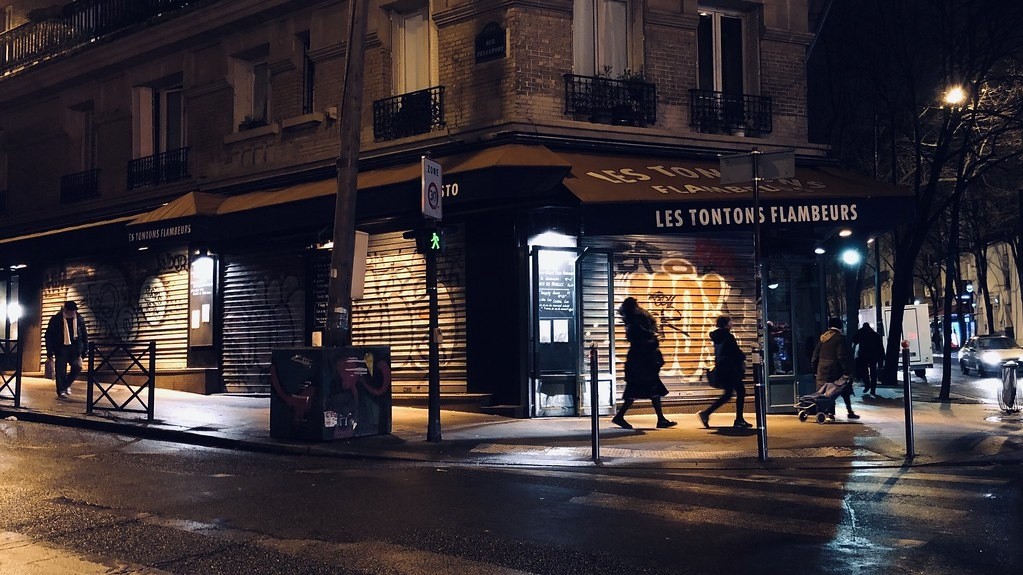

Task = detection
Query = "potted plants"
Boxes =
[576,63,656,129]
[238,114,269,131]
[695,94,773,138]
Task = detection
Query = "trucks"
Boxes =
[857,303,933,376]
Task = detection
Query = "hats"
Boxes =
[622,297,637,310]
[64,300,79,311]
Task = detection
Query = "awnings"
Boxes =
[552,151,925,235]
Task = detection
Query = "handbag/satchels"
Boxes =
[706,365,732,389]
[798,393,828,402]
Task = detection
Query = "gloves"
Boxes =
[81,350,88,359]
[843,374,850,380]
[47,355,53,362]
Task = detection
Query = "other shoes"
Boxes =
[58,392,69,401]
[828,414,836,421]
[870,392,876,398]
[863,385,871,394]
[847,413,860,420]
[66,386,72,395]
[657,420,677,428]
[612,416,632,429]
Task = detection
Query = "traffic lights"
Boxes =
[416,229,443,254]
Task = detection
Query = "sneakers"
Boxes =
[733,419,752,428]
[698,410,710,429]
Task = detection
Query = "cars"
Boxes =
[958,335,1023,378]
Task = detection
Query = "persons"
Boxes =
[813,319,861,420]
[697,315,752,428]
[610,296,678,429]
[854,322,887,394]
[45,300,88,398]
[932,329,942,354]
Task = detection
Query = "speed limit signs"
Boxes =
[428,183,439,209]
